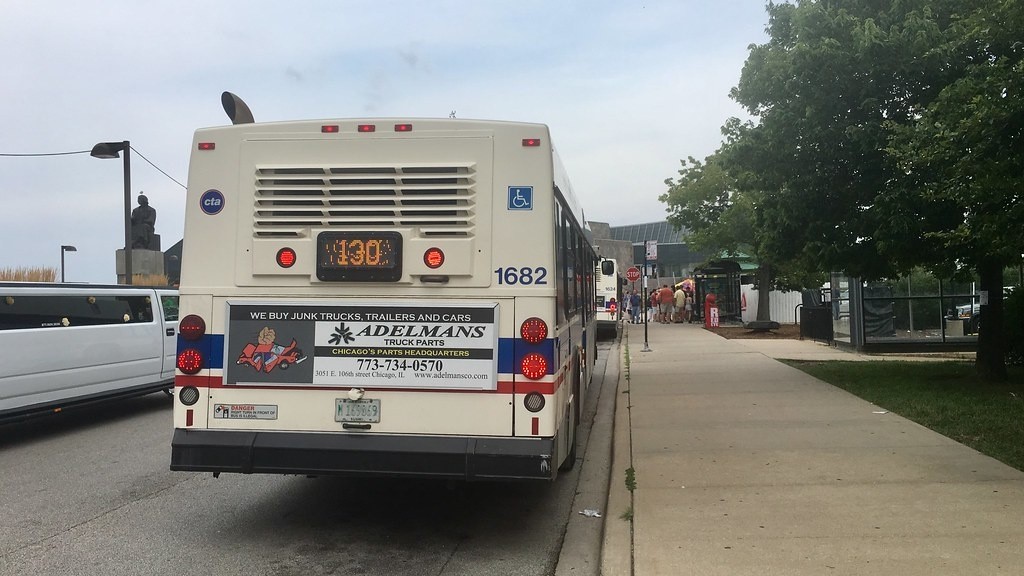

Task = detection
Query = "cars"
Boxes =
[956,303,981,333]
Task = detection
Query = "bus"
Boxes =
[594,259,628,338]
[169,89,614,482]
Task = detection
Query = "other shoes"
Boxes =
[688,320,692,323]
[636,323,640,324]
[631,321,634,324]
[661,321,665,324]
[674,320,683,323]
[666,321,671,324]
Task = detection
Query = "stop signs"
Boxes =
[625,267,641,282]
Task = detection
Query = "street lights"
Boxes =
[61,245,77,282]
[89,140,134,284]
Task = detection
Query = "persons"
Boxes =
[658,284,674,324]
[621,289,629,311]
[648,288,661,322]
[680,285,694,323]
[674,286,685,323]
[131,195,156,248]
[630,289,641,324]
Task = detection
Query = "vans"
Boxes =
[0,280,180,424]
